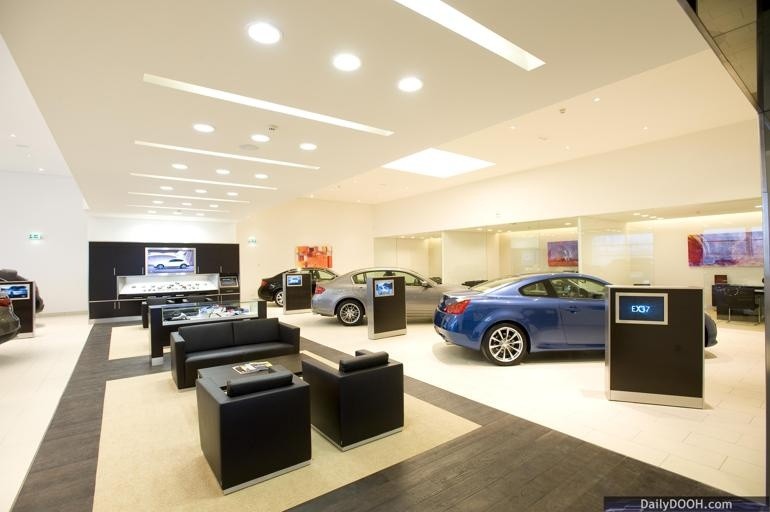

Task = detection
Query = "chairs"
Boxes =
[196,347,404,496]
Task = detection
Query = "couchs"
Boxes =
[170,317,300,389]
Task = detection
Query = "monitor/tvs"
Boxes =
[616,292,669,325]
[287,275,302,287]
[374,279,395,297]
[0,283,30,300]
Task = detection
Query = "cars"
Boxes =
[0,269,44,344]
[258,268,339,307]
[311,267,471,326]
[433,271,717,366]
